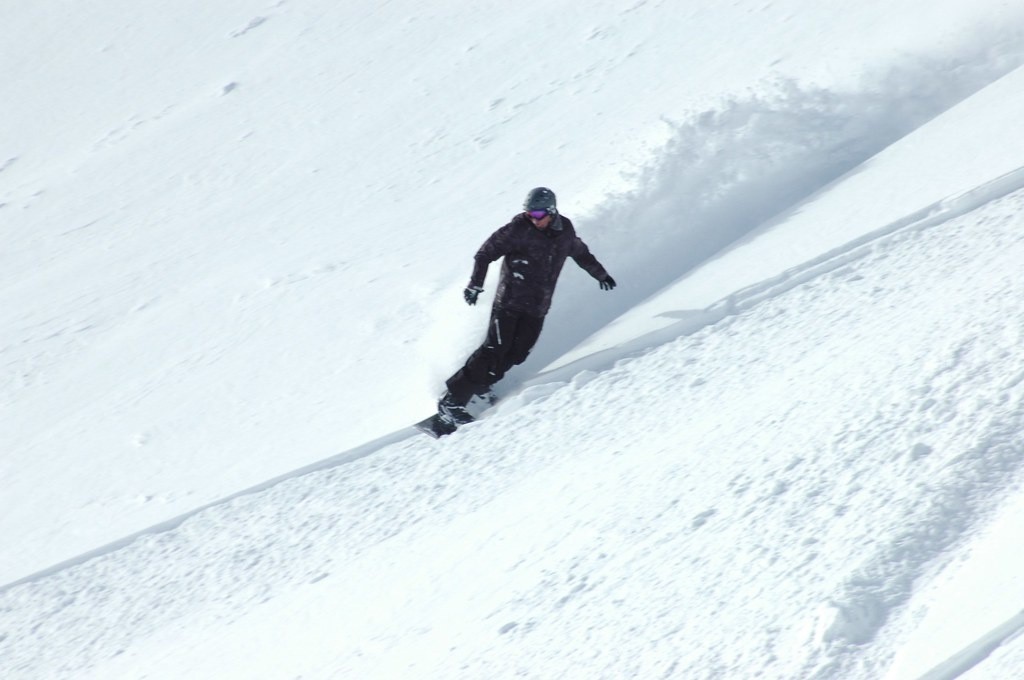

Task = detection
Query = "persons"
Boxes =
[437,188,617,425]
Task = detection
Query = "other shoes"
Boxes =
[437,398,471,424]
[476,390,497,405]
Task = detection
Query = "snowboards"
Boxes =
[412,379,499,441]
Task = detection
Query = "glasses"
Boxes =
[526,209,548,219]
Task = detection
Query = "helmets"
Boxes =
[523,186,556,214]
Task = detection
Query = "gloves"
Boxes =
[600,276,616,291]
[465,285,484,306]
